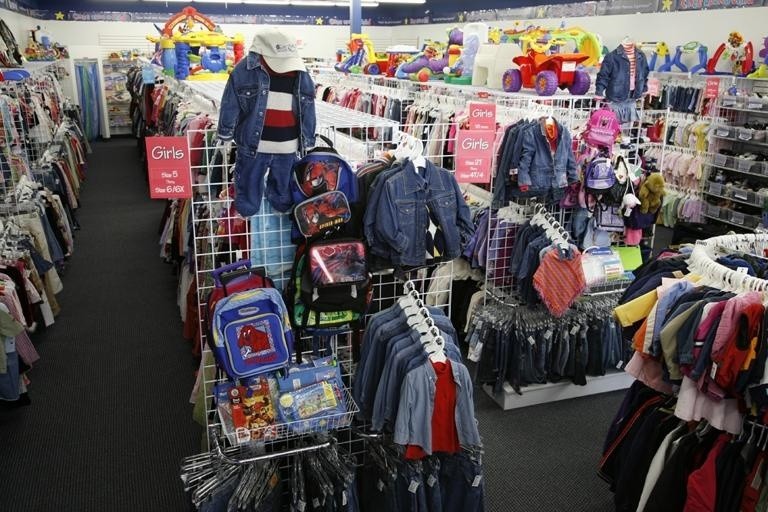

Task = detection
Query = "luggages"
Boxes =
[205,260,274,373]
[207,265,296,383]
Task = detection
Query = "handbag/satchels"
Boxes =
[594,199,624,234]
[604,154,637,205]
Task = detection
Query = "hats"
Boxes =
[247,27,308,75]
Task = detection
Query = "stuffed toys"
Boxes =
[638,172,666,216]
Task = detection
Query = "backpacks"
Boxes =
[288,130,359,245]
[302,236,375,315]
[290,249,362,329]
[584,107,620,147]
[584,157,615,189]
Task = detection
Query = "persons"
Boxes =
[594,40,649,95]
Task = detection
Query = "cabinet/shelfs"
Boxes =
[701,77,768,232]
[98,57,141,139]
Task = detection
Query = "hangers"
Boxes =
[659,231,768,311]
[526,203,577,260]
[655,392,767,455]
[312,73,589,169]
[634,76,724,204]
[379,281,464,374]
